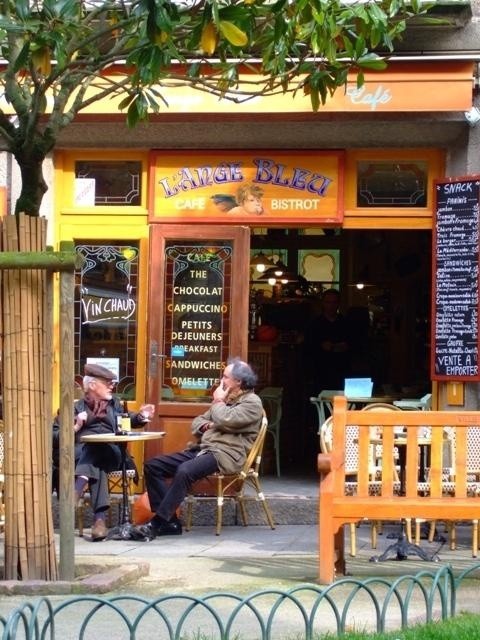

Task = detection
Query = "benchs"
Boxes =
[315,395,480,584]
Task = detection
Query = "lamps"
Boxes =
[249,224,380,293]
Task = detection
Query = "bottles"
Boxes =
[123,400,128,417]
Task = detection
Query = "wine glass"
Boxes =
[141,403,153,423]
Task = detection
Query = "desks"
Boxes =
[82,431,171,539]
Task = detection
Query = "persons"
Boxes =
[211,194,239,213]
[227,184,270,218]
[125,360,263,542]
[51,364,156,543]
[302,289,357,390]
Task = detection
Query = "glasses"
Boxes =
[96,381,119,386]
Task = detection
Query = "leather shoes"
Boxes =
[140,519,182,536]
[92,520,108,539]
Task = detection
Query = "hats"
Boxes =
[84,364,118,380]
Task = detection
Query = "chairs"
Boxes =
[309,385,479,562]
[68,371,288,454]
[185,409,277,536]
